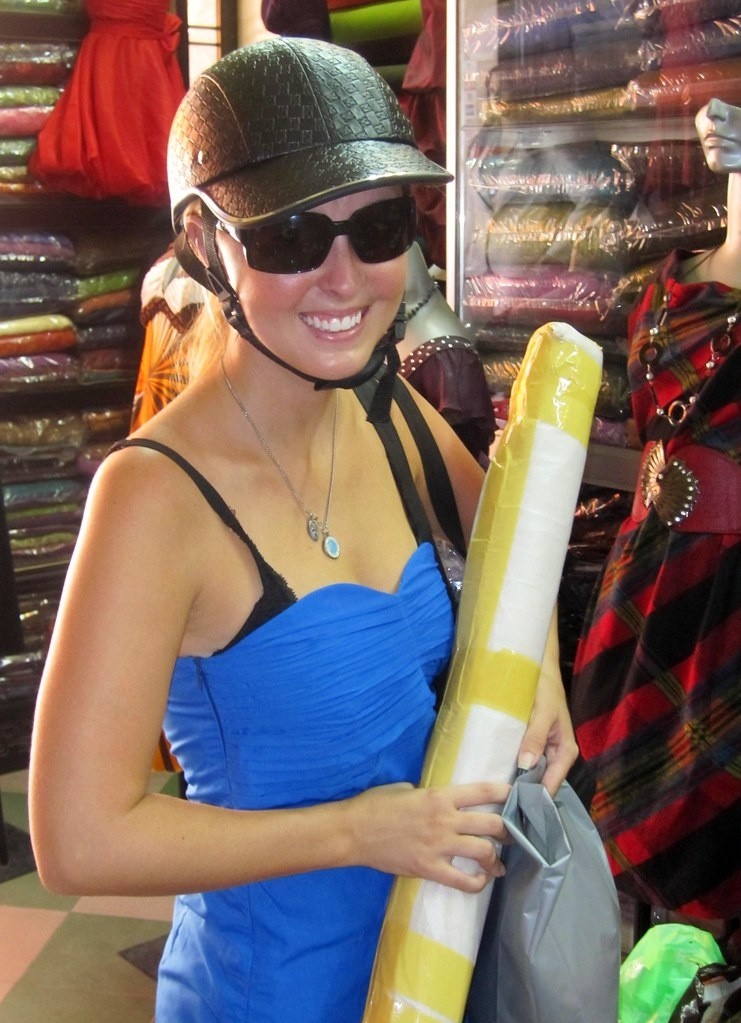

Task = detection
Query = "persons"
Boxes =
[30,38,582,1023]
[465,66,588,278]
[575,94,741,947]
[33,0,498,464]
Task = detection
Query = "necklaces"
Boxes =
[221,357,339,559]
[640,244,741,426]
[405,282,438,320]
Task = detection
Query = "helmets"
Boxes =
[166,38,454,238]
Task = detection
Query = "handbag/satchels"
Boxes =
[465,753,621,1023]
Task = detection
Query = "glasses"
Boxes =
[205,196,416,274]
[683,78,740,114]
